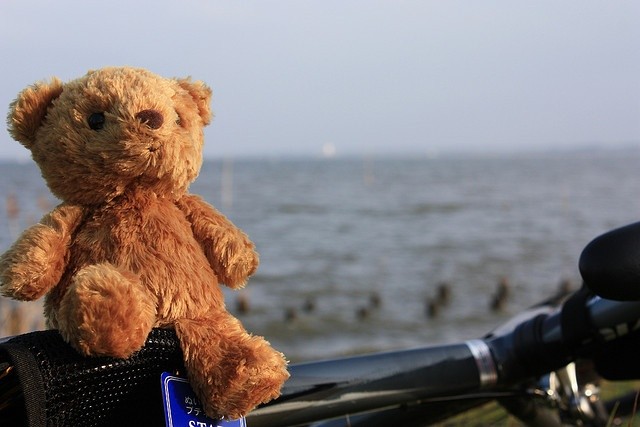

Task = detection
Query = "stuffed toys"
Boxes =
[0,66,289,420]
